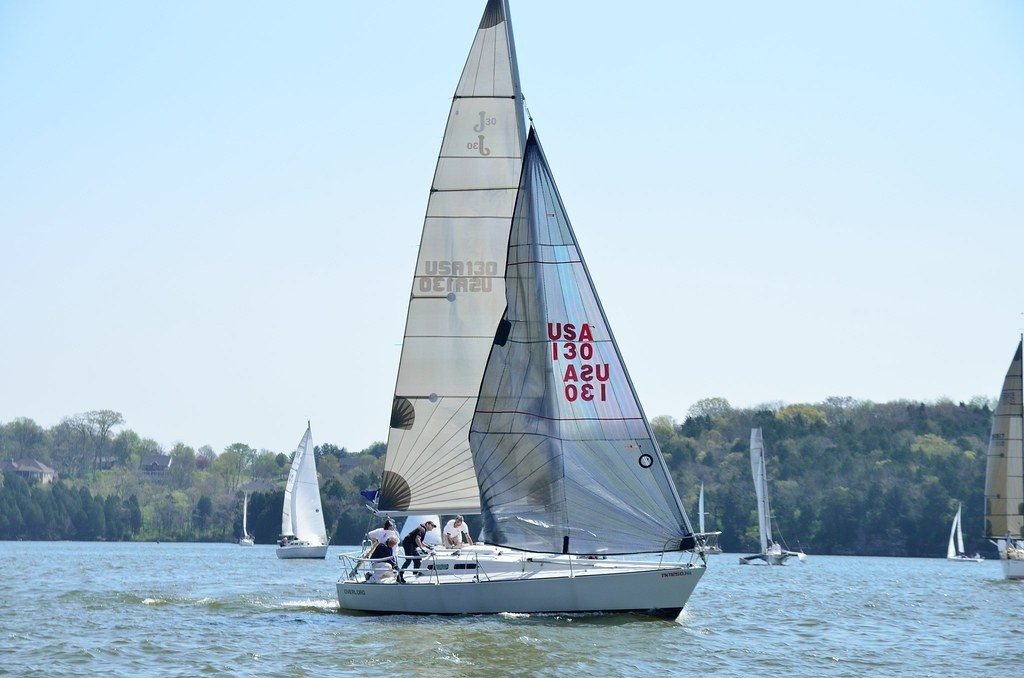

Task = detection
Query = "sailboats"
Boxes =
[946,503,983,563]
[238,492,255,547]
[275,420,333,559]
[693,482,723,553]
[333,0,707,620]
[740,423,807,567]
[983,332,1024,579]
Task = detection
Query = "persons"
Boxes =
[368,520,401,548]
[444,515,473,549]
[396,521,437,584]
[370,537,398,566]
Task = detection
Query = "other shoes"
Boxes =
[413,572,422,576]
[397,576,406,583]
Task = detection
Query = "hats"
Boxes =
[427,520,436,528]
